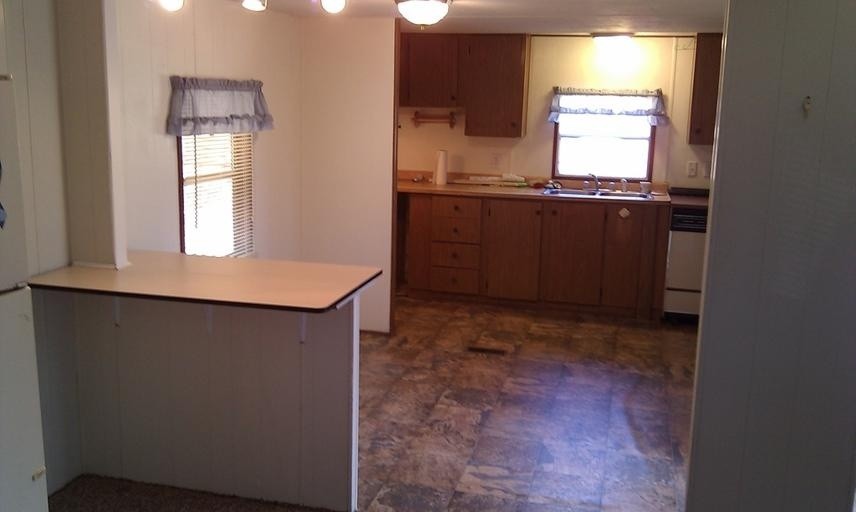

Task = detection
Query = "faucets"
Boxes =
[587,172,603,190]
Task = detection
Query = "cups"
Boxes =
[639,181,651,194]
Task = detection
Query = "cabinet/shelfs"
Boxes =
[688,33,724,145]
[399,33,531,138]
[403,193,669,325]
[663,193,709,316]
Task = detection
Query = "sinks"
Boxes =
[597,191,655,201]
[542,187,597,199]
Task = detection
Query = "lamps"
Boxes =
[394,0,454,31]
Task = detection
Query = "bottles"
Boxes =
[620,178,627,193]
